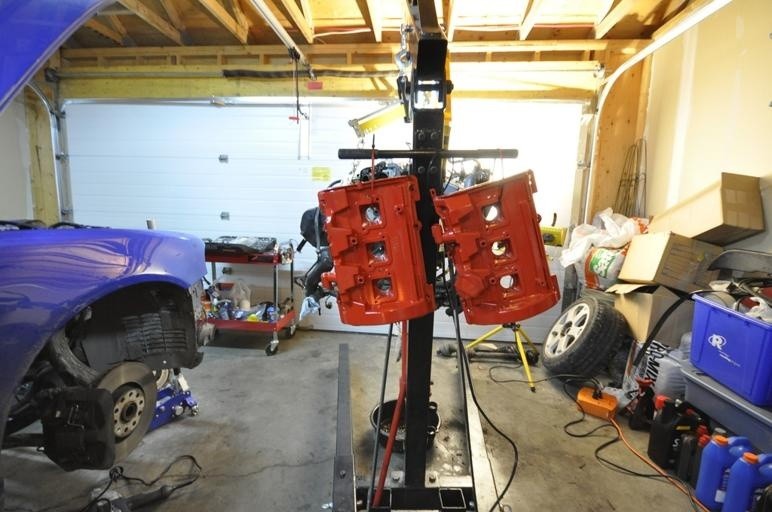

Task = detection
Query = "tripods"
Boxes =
[464,322,541,393]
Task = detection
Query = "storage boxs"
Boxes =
[607,283,694,352]
[618,230,724,292]
[644,170,767,247]
[688,285,771,409]
[678,367,770,460]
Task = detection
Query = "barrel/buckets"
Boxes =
[695,434,754,512]
[647,398,712,471]
[720,452,772,512]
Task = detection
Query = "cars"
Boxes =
[1,1,218,472]
